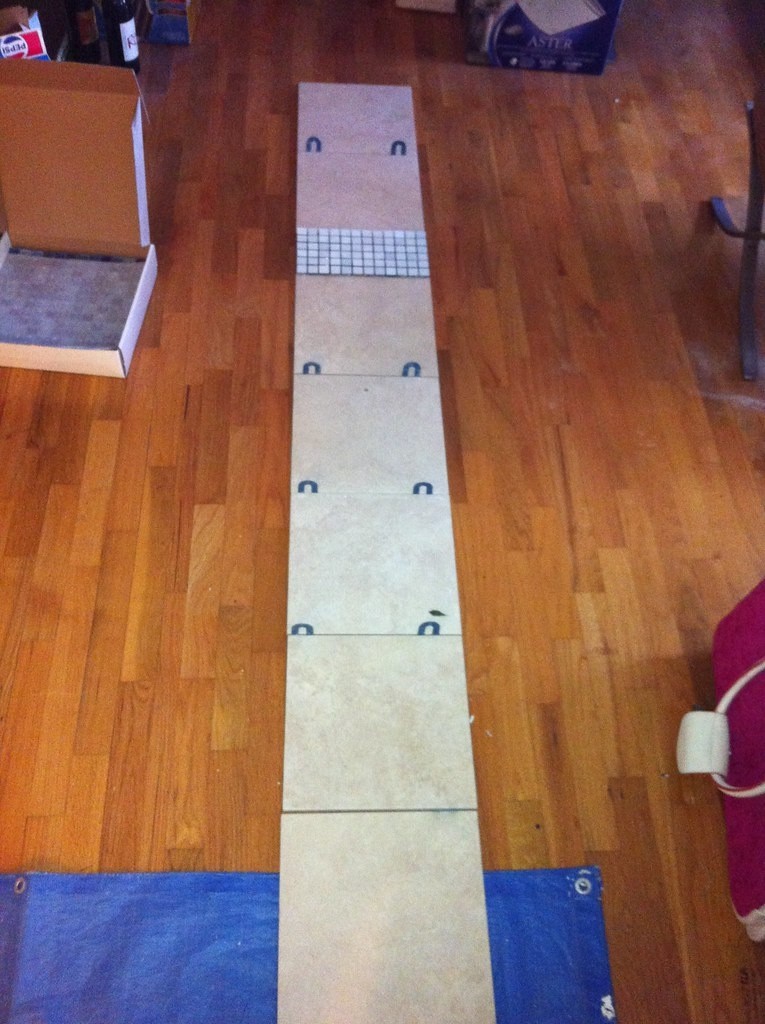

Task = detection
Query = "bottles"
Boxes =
[103,0,139,76]
[63,0,101,65]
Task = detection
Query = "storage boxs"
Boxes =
[0,58,159,378]
[0,6,51,61]
[146,0,199,43]
[465,0,623,76]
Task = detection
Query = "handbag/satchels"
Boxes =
[676,575,765,943]
